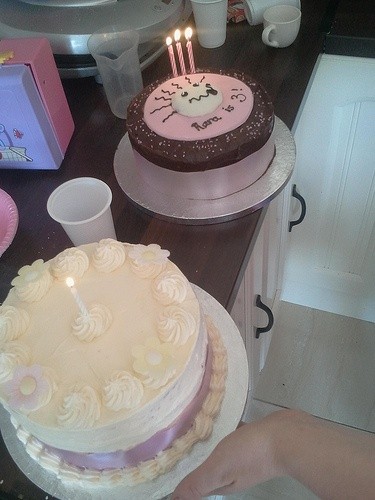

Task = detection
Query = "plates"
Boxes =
[0,188,19,260]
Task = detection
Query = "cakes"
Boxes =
[126,65,274,200]
[0,238,228,491]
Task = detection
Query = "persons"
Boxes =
[170,409,375,500]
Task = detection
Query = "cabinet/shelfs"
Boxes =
[0,0,375,500]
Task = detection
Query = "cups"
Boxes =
[191,0,228,49]
[47,177,118,247]
[244,0,301,26]
[262,5,301,48]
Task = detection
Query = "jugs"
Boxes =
[87,26,144,120]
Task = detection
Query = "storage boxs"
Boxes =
[0,37,75,170]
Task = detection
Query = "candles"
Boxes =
[185,26,196,74]
[166,36,179,78]
[174,28,187,76]
